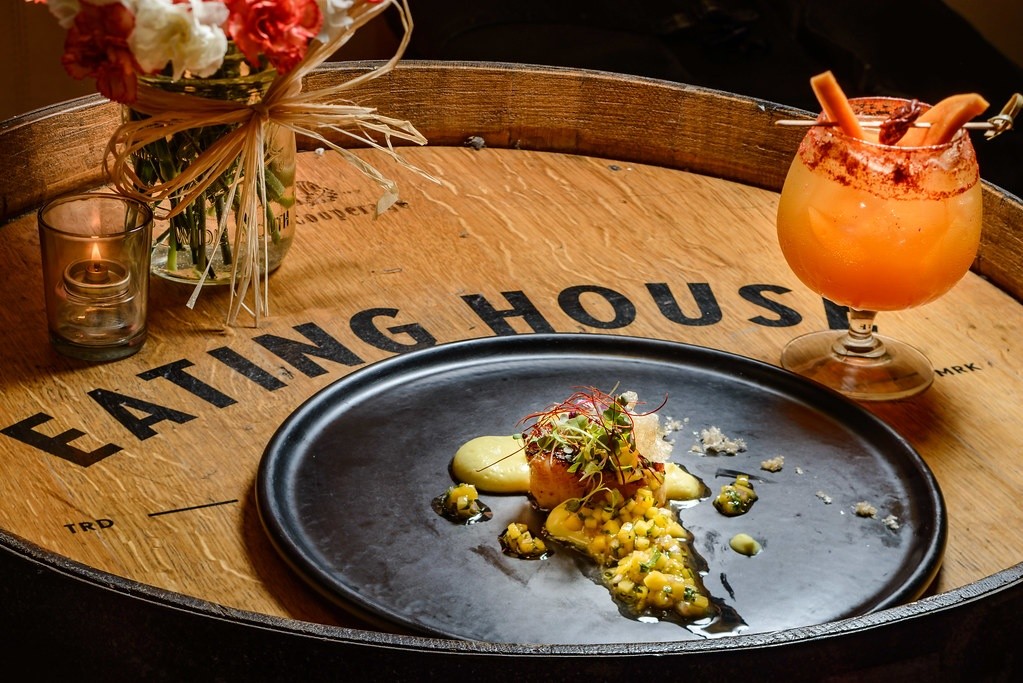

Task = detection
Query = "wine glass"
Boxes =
[777,97,983,401]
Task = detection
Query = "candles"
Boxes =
[39,194,155,360]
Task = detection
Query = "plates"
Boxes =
[256,333,947,645]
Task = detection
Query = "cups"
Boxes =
[36,192,152,362]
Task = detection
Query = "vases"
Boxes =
[125,68,304,287]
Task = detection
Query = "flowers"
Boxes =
[25,0,384,106]
